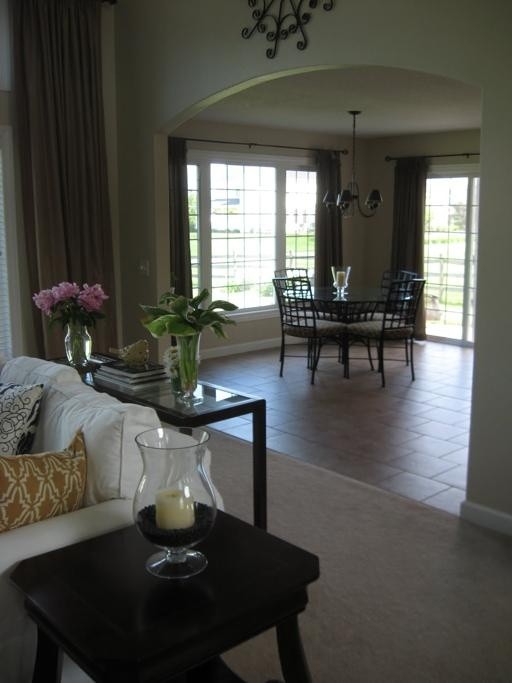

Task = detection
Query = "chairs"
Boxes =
[272,267,426,388]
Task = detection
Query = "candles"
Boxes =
[153,486,196,532]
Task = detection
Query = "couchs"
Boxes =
[0,355,163,682]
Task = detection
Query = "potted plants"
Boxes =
[135,288,240,405]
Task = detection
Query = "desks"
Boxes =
[75,351,268,534]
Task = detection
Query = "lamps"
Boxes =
[321,109,383,219]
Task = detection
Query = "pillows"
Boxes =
[0,429,87,533]
[0,382,45,457]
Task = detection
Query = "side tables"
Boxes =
[5,499,321,683]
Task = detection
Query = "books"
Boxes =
[93,359,171,390]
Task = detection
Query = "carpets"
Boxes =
[150,418,511,683]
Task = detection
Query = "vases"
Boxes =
[169,366,182,393]
[64,320,93,366]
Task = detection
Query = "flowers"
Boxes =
[32,279,110,330]
[161,344,185,380]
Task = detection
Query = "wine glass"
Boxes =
[330,264,352,301]
[134,424,220,581]
